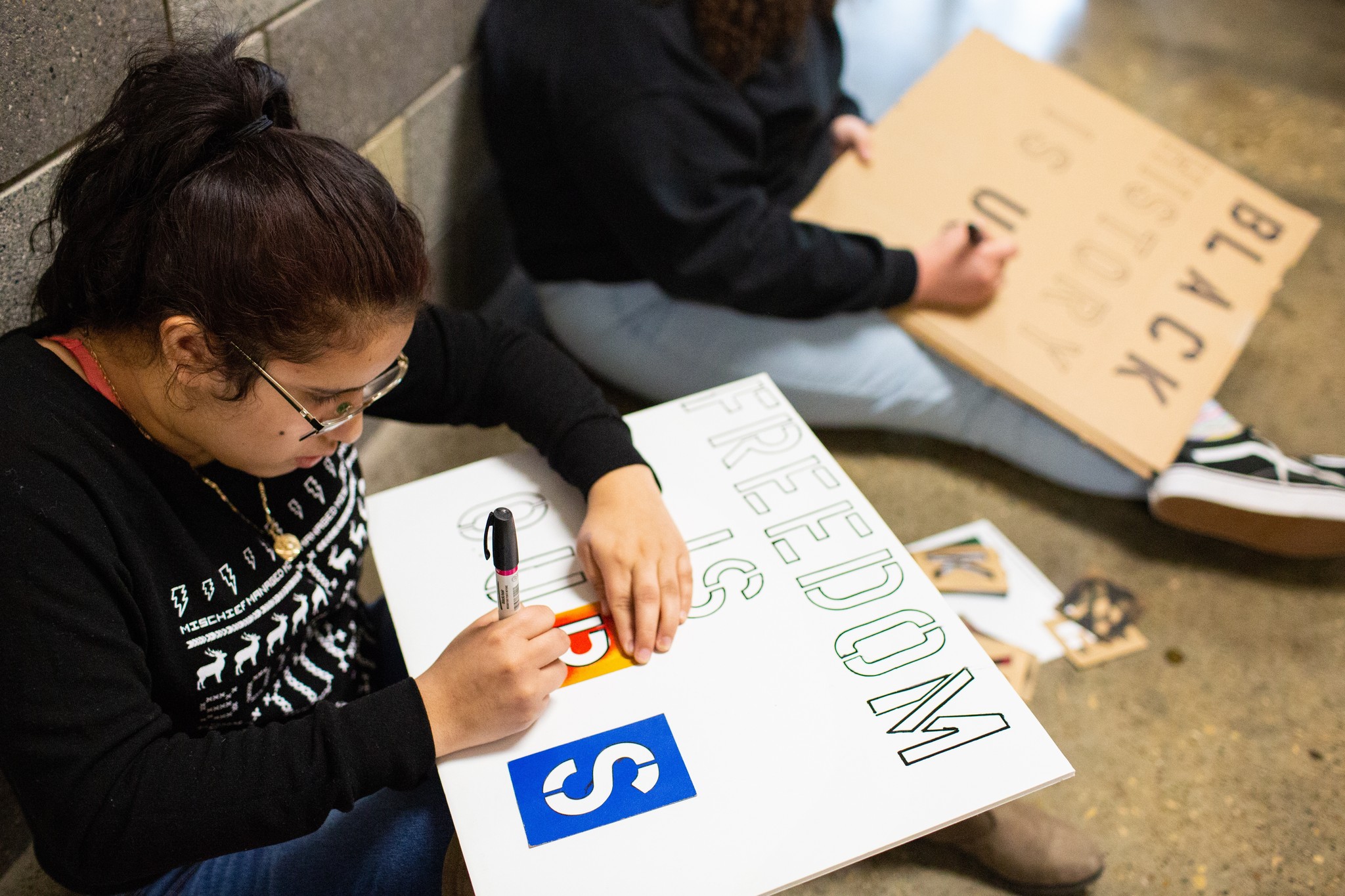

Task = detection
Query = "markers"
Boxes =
[483,507,521,625]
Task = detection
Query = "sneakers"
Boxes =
[1148,425,1345,562]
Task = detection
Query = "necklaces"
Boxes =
[65,322,305,566]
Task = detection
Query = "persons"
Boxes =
[442,0,1345,560]
[1,28,1105,896]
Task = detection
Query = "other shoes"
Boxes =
[922,801,1104,893]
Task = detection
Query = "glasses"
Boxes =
[217,335,408,442]
